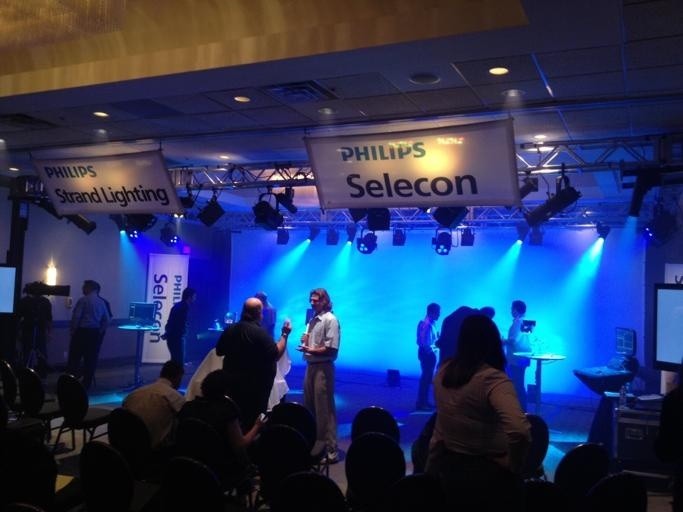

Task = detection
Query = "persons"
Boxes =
[1,280,683,512]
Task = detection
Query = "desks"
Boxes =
[118,324,160,392]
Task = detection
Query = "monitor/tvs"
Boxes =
[652,284,683,374]
[616,327,636,356]
[129,302,157,323]
[0,263,17,316]
